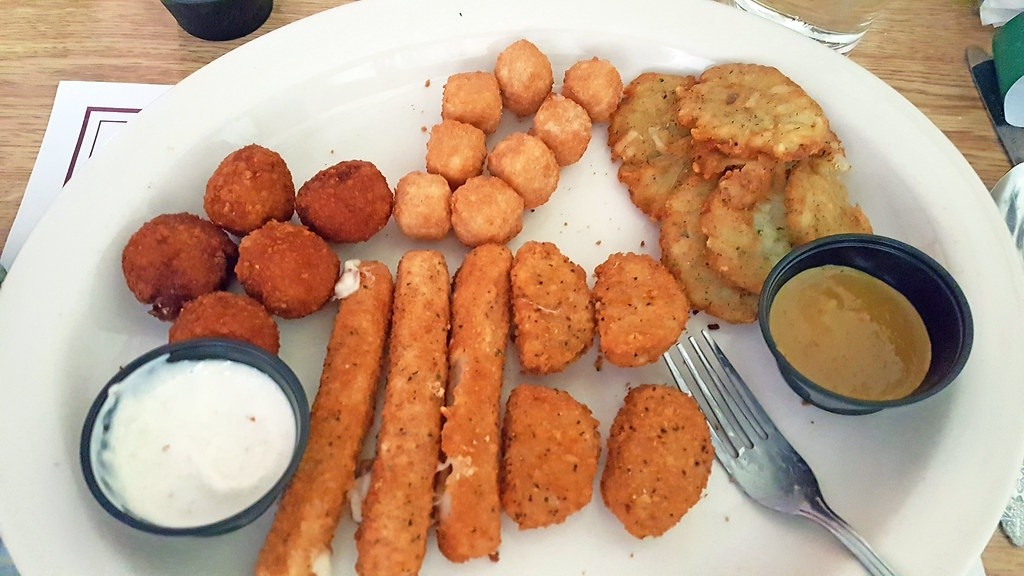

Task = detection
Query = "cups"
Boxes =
[715,0,887,59]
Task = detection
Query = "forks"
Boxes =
[664,328,896,576]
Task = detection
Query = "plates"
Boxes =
[0,0,1024,576]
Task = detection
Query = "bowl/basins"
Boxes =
[758,232,974,416]
[80,338,309,537]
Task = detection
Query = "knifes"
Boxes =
[965,45,1024,167]
